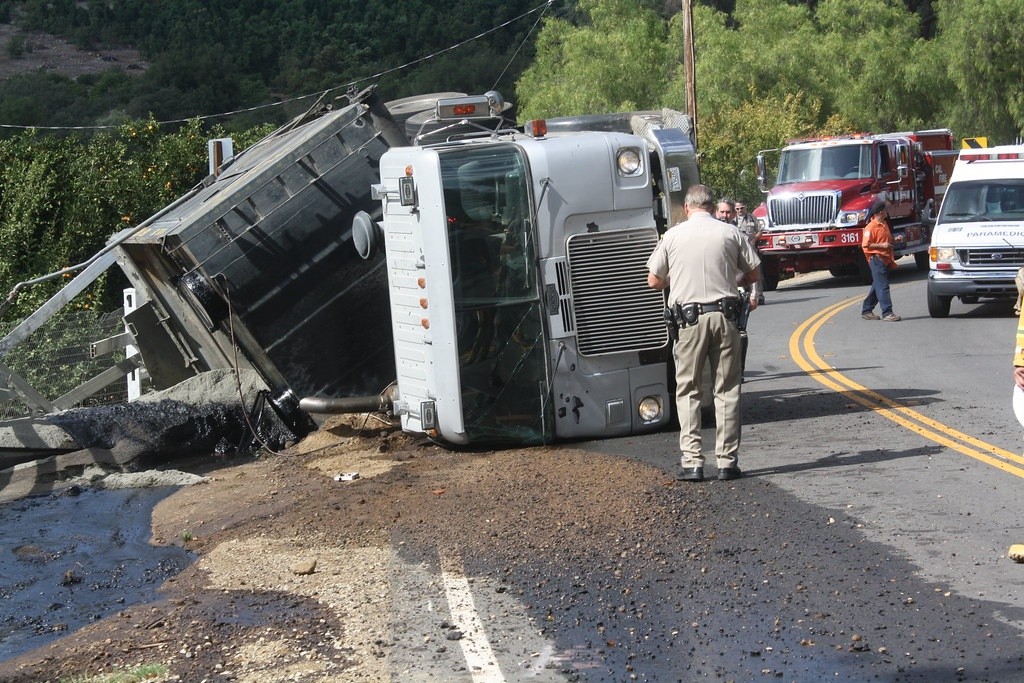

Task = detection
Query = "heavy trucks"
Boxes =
[108,73,702,449]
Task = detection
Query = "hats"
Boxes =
[870,202,886,214]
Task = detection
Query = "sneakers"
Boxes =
[882,313,901,322]
[861,312,880,320]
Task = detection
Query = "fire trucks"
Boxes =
[751,129,955,292]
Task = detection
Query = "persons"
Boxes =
[715,198,763,382]
[646,184,761,480]
[861,202,904,321]
[1009,265,1024,562]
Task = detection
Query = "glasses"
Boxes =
[735,206,746,210]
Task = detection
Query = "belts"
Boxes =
[696,300,722,314]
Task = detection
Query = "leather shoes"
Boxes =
[717,466,742,481]
[678,466,705,481]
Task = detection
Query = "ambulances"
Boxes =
[924,143,1024,320]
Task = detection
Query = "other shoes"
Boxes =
[758,297,766,305]
